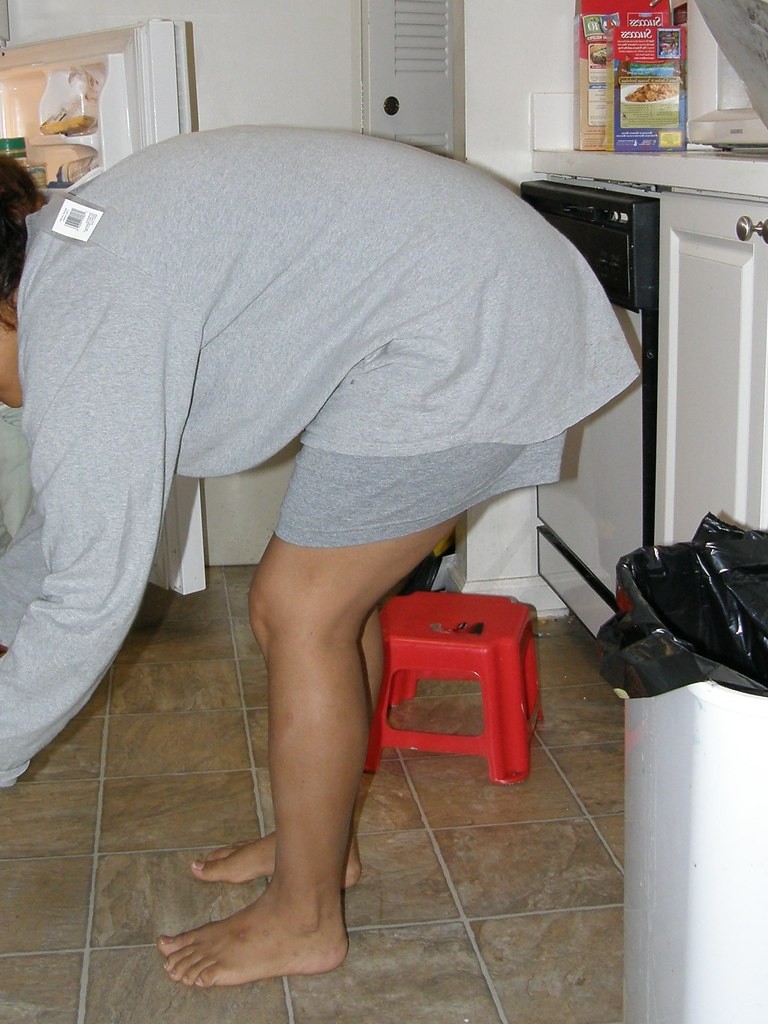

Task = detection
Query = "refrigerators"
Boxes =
[0,1,207,595]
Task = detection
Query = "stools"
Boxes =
[362,589,546,786]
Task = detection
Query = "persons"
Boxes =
[1,126,641,990]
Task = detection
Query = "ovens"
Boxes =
[519,177,659,639]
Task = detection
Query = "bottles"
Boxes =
[21,162,47,189]
[0,138,28,167]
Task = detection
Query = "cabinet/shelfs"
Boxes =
[651,187,768,545]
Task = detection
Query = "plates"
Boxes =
[621,86,679,105]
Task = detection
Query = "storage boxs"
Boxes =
[572,0,690,152]
